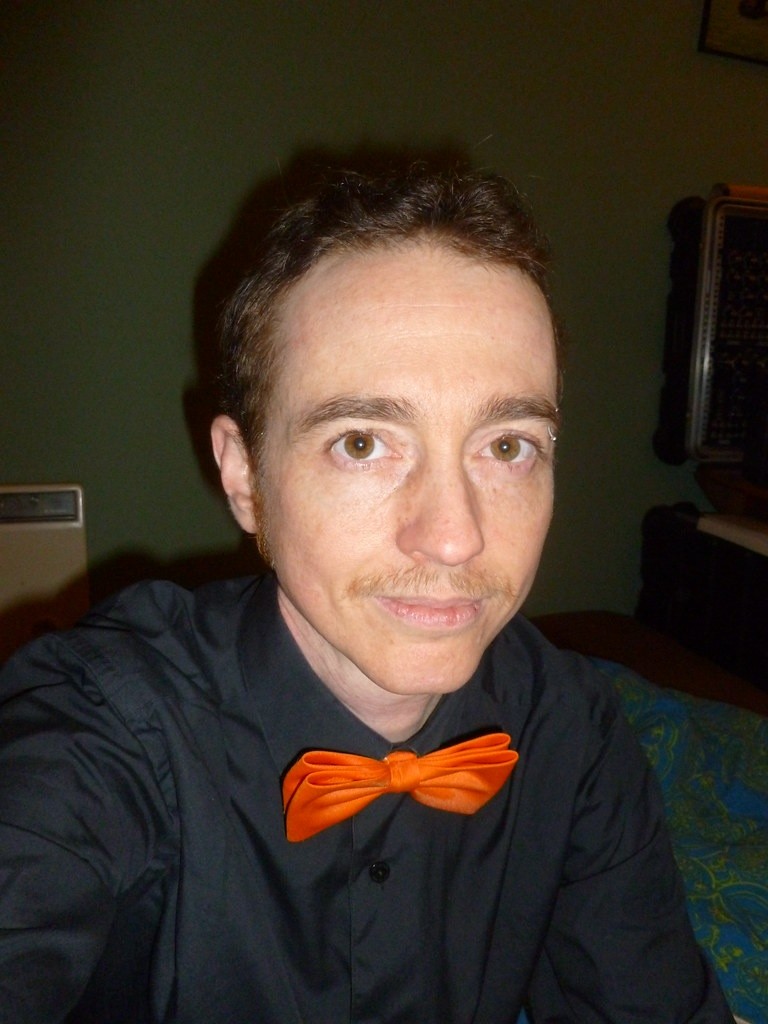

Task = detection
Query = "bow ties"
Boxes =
[281,732,519,845]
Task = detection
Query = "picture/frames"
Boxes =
[698,0,768,64]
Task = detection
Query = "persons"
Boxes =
[0,150,737,1024]
